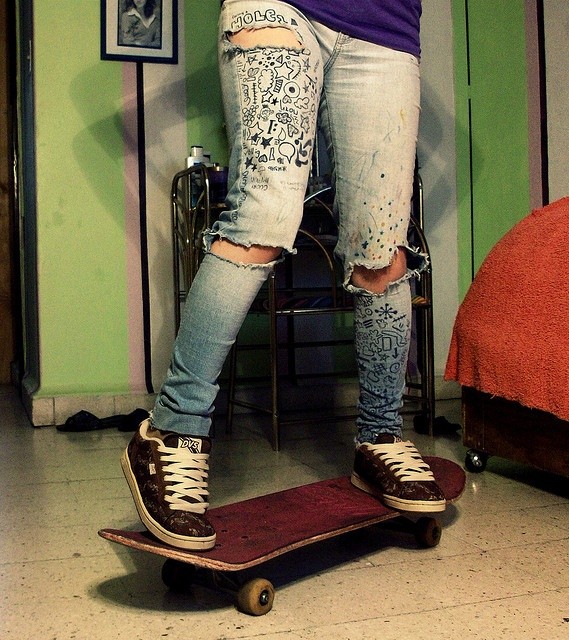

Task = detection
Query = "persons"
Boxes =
[118,0,446,551]
[121,0,160,46]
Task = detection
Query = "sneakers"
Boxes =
[350,433,446,512]
[119,416,217,552]
[413,413,462,433]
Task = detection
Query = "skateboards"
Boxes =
[97,455,467,616]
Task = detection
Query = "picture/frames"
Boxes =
[99,1,179,64]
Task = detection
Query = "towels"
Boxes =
[442,197,569,421]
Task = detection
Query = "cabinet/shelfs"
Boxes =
[171,162,434,451]
